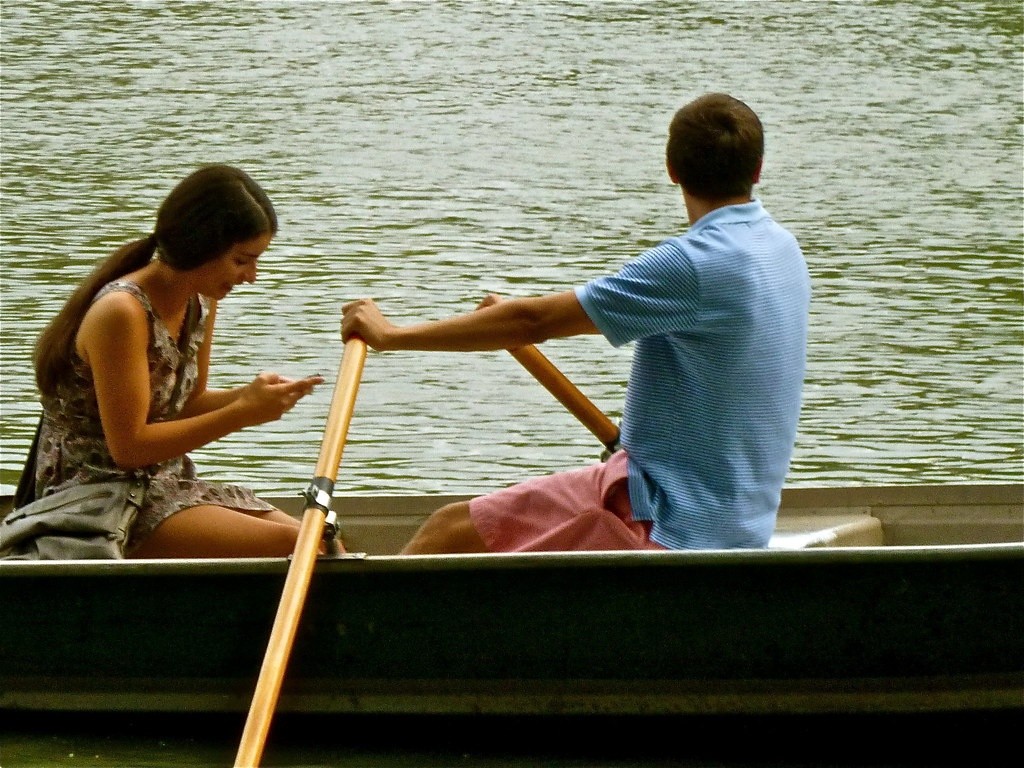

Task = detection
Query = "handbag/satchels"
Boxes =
[0,480,146,562]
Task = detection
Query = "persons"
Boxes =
[341,92,811,557]
[12,164,346,559]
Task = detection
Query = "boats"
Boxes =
[0,476,1024,768]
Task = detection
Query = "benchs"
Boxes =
[771,512,894,546]
